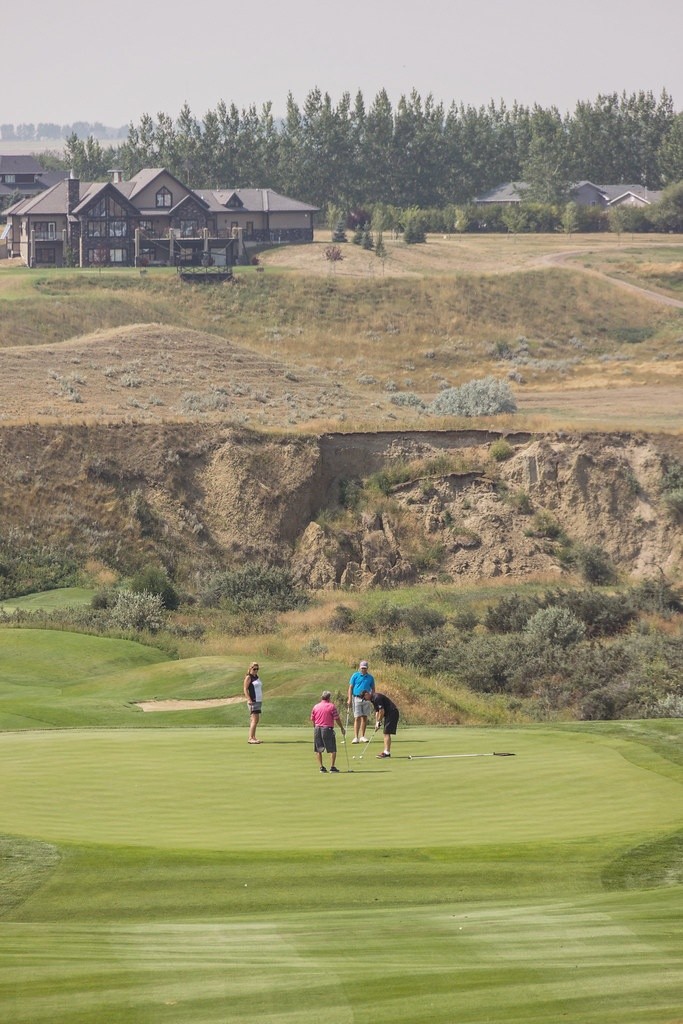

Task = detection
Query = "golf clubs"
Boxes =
[341,707,351,744]
[342,733,354,773]
[360,731,375,756]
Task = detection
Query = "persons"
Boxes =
[311,691,346,774]
[244,662,263,744]
[361,690,399,756]
[347,661,375,743]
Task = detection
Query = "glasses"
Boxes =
[252,667,259,671]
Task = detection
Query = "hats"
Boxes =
[359,661,369,669]
[360,689,367,702]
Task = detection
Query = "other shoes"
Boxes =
[248,738,264,744]
[359,737,369,742]
[319,766,327,773]
[330,767,339,773]
[351,737,359,744]
[375,753,391,759]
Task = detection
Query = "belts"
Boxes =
[354,695,362,698]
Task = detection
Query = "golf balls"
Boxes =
[352,755,356,759]
[359,756,362,759]
